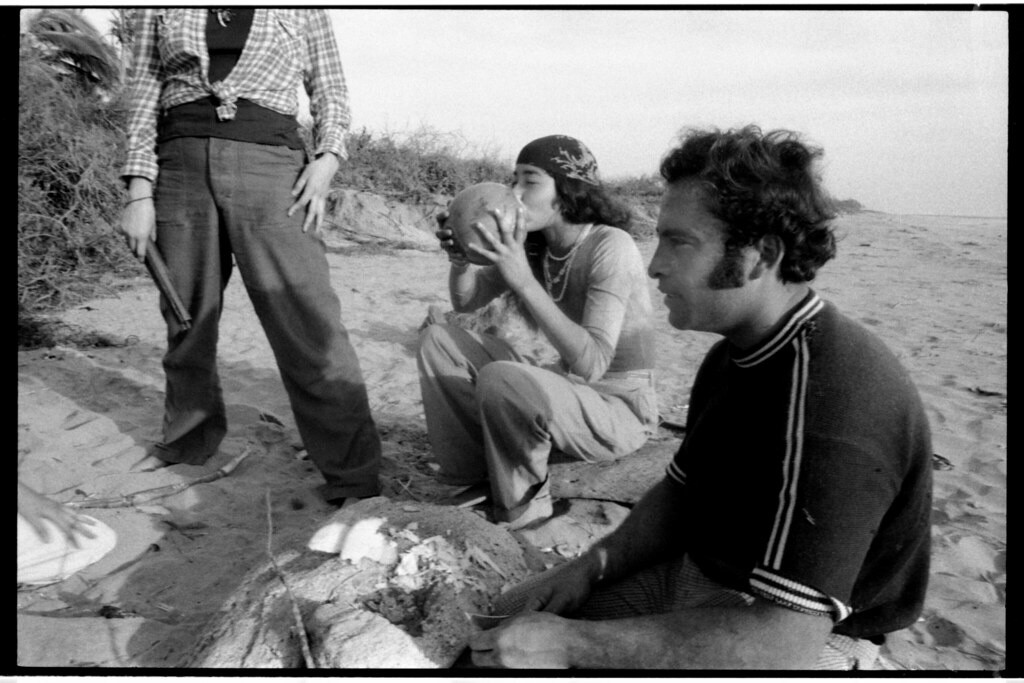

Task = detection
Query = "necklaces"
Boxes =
[543,222,593,303]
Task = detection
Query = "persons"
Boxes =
[120,7,413,506]
[414,136,658,530]
[473,123,932,671]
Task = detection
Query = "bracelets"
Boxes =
[124,195,155,208]
[447,257,470,269]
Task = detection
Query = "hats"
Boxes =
[517,134,600,187]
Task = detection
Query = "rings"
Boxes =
[486,604,496,616]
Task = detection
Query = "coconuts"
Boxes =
[447,181,527,266]
